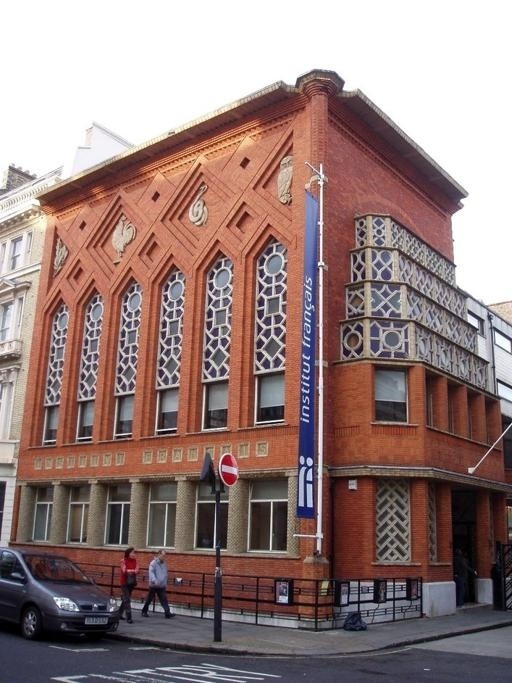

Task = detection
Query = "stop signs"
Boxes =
[219,453,239,487]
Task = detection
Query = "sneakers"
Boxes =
[120,616,132,623]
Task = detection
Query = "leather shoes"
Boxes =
[165,613,175,618]
[142,612,148,617]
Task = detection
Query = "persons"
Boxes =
[118,547,140,623]
[453,548,478,609]
[141,549,176,619]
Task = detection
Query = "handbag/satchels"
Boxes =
[126,574,137,586]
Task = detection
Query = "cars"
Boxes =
[0,546,120,640]
